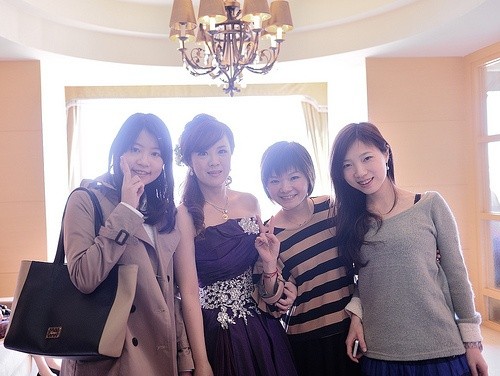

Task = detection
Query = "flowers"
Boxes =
[237,217,259,235]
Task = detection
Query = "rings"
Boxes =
[288,306,291,310]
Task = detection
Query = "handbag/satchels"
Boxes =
[3,187,138,359]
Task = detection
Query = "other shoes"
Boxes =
[36,373,41,376]
[49,366,60,376]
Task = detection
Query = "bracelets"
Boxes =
[262,267,278,278]
[463,341,483,351]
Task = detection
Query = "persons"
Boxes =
[330,123,489,376]
[173,113,300,376]
[59,112,195,376]
[0,304,62,376]
[250,140,441,376]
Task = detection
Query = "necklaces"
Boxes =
[289,217,310,227]
[204,188,229,219]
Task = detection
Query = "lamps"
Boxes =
[170,0,293,96]
